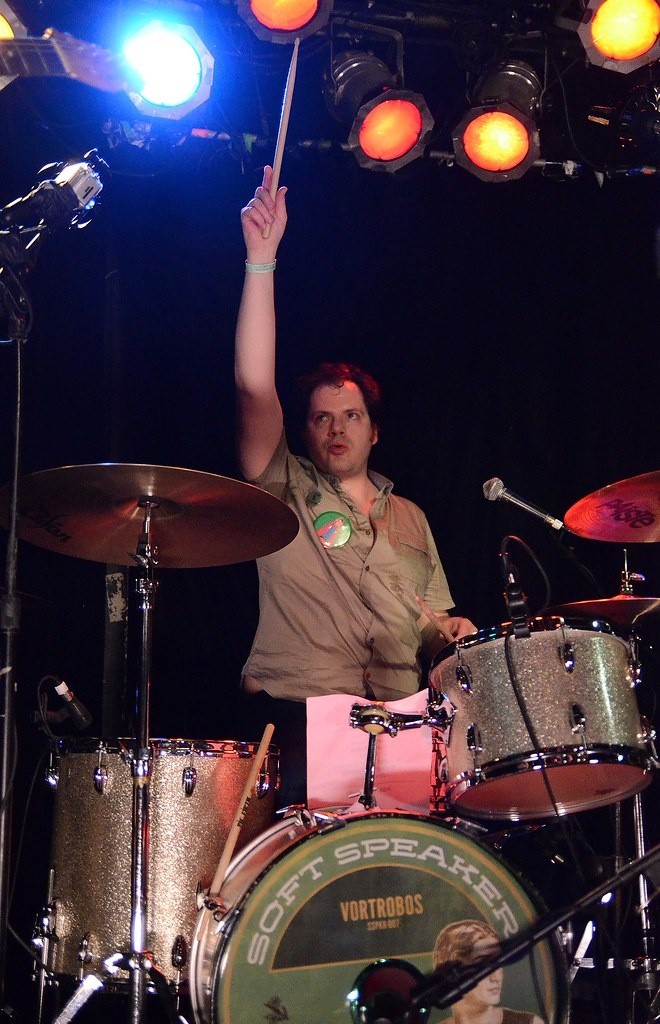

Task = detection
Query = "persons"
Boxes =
[235,165,479,806]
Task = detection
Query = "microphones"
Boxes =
[498,550,520,596]
[482,476,564,531]
[364,964,468,1024]
[53,680,93,729]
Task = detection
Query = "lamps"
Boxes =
[577,0,660,74]
[450,0,560,183]
[112,0,214,119]
[0,0,42,38]
[237,0,334,45]
[322,17,435,173]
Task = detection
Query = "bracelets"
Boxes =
[245,259,276,273]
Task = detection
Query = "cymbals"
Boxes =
[563,471,660,543]
[0,463,301,569]
[558,594,660,623]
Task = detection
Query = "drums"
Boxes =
[189,804,572,1024]
[426,616,660,821]
[35,737,280,997]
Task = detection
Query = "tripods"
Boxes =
[51,505,188,1024]
[570,791,660,1024]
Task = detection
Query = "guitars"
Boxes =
[0,26,146,92]
